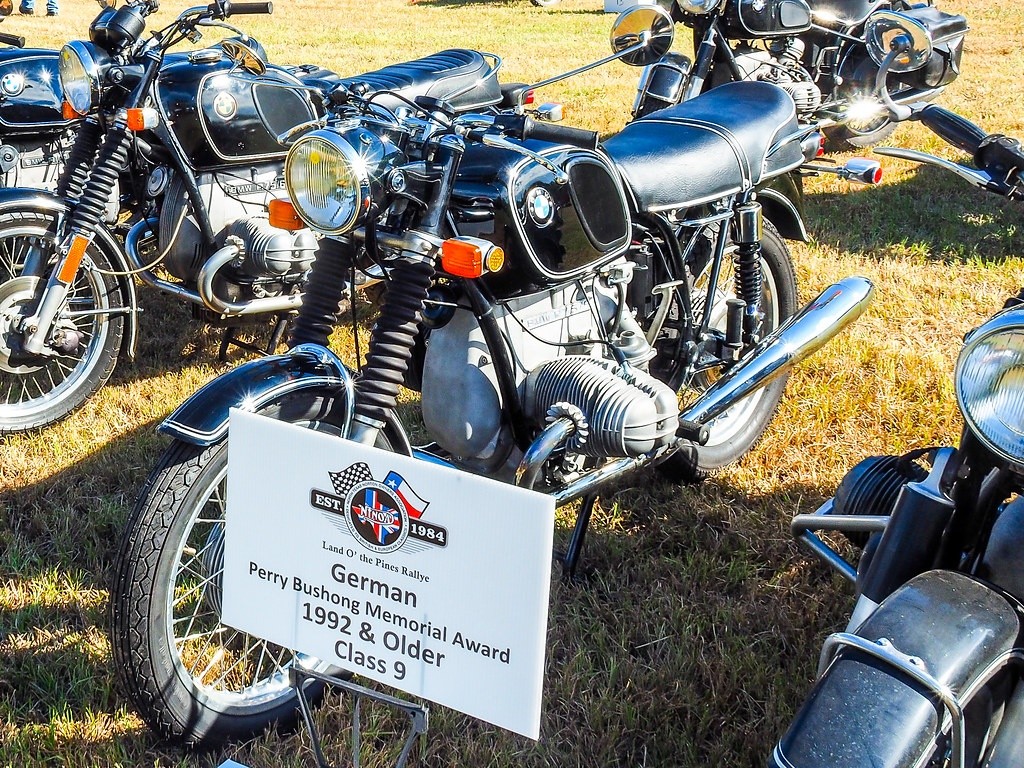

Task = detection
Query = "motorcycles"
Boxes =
[0,0,877,758]
[622,0,971,168]
[763,105,1024,768]
